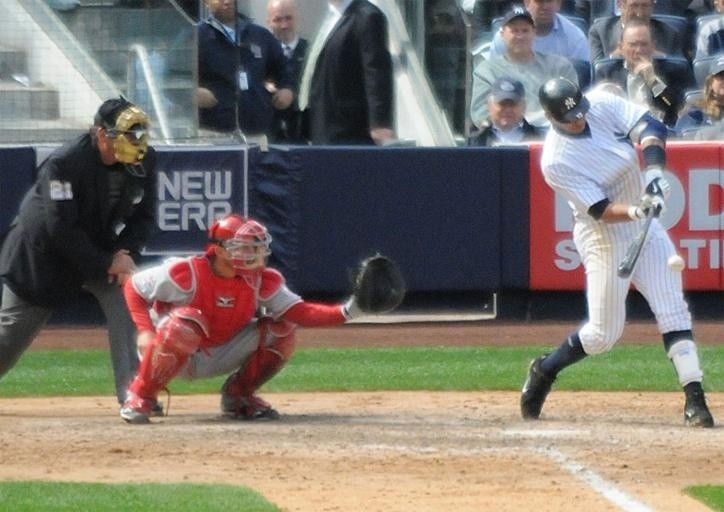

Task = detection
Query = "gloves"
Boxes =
[629,176,670,220]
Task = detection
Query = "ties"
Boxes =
[298,12,333,111]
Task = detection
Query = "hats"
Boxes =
[493,79,523,103]
[503,7,534,25]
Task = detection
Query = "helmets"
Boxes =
[539,77,590,123]
[205,216,273,274]
[95,99,151,165]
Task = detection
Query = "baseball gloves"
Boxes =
[355,257,404,313]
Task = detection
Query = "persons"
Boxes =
[463,76,541,146]
[583,1,684,79]
[0,95,171,418]
[111,214,410,423]
[488,0,590,80]
[517,75,715,428]
[160,1,297,144]
[467,8,581,149]
[592,18,694,132]
[265,1,311,144]
[692,0,723,53]
[292,1,399,147]
[673,56,723,141]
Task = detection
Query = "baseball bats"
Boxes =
[617,207,655,278]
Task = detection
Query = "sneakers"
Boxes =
[521,355,551,420]
[121,401,164,424]
[221,395,280,419]
[685,400,713,427]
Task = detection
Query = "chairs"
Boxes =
[491,0,724,140]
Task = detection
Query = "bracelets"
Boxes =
[641,145,667,169]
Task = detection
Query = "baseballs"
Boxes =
[667,256,684,271]
